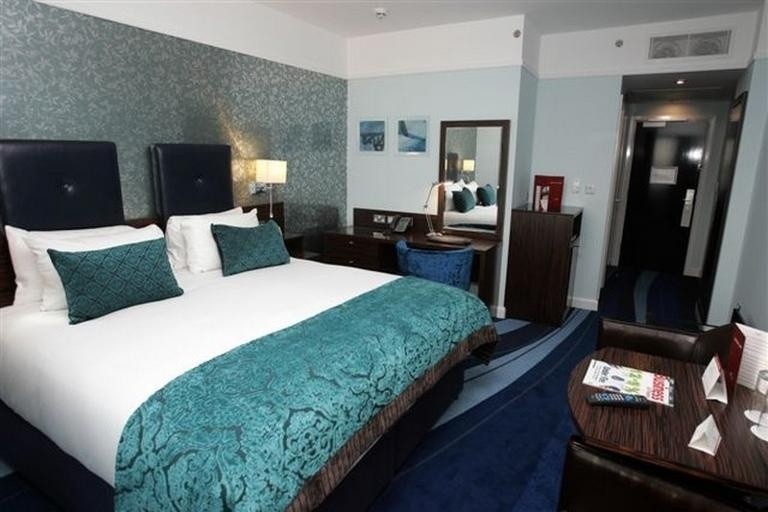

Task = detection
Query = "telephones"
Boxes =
[389,214,412,233]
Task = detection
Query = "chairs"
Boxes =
[598,317,697,363]
[556,435,768,512]
[397,239,479,299]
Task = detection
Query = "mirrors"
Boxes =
[437,120,510,243]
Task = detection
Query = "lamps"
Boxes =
[254,159,287,221]
[423,180,454,241]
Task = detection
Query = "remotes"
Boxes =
[587,392,649,408]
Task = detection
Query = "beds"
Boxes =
[0,139,500,512]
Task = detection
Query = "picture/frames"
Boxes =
[396,115,429,155]
[358,118,387,154]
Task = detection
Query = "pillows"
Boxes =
[4,224,139,312]
[179,209,259,274]
[46,238,183,324]
[210,220,290,277]
[444,179,496,213]
[166,206,248,277]
[21,223,176,312]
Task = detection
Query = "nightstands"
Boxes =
[283,231,320,261]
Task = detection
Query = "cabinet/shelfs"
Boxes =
[505,203,584,327]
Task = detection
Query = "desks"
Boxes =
[309,226,498,313]
[567,347,767,500]
